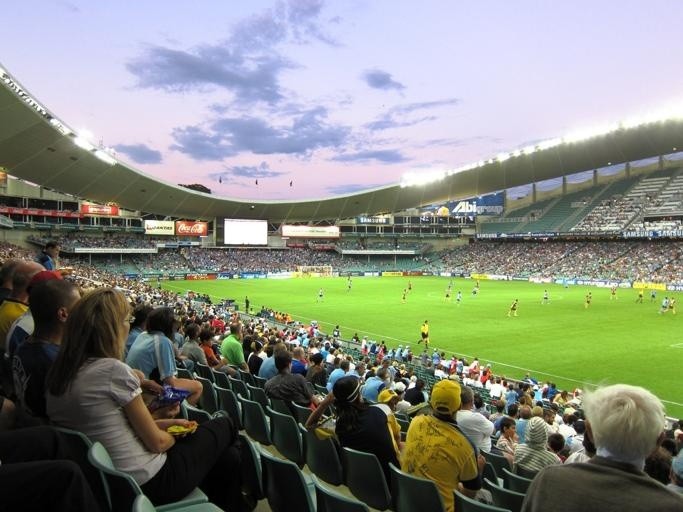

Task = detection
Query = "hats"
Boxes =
[254,339,264,347]
[25,270,64,295]
[377,389,398,404]
[431,378,463,416]
[394,381,407,392]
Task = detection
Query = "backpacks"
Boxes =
[314,427,341,447]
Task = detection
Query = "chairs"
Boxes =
[0,342,683,512]
[29,162,683,279]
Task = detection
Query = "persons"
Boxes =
[0,191,683,512]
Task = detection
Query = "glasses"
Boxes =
[124,316,136,323]
